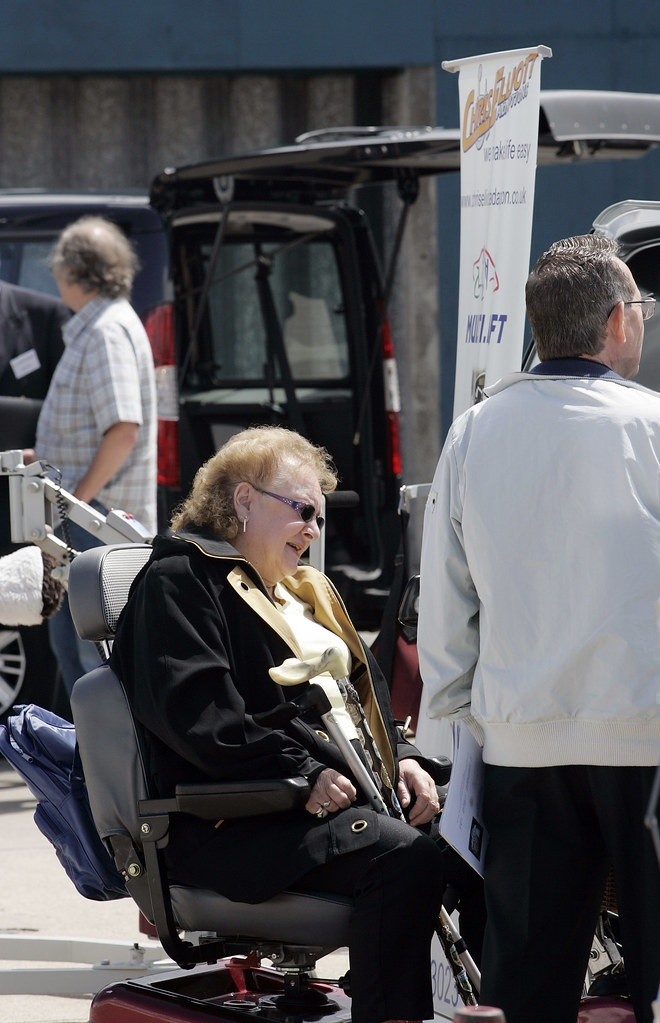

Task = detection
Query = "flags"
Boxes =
[451,53,545,431]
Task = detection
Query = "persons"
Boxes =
[109,428,488,1023]
[415,235,660,1023]
[22,218,158,702]
[0,279,78,715]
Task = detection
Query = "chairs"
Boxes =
[68,543,351,969]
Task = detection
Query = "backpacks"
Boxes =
[0,701,131,902]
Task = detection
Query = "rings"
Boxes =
[314,806,325,819]
[324,798,332,808]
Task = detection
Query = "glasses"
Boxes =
[255,488,325,532]
[606,297,656,321]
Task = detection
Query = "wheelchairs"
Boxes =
[62,537,504,1023]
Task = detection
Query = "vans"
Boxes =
[0,93,659,730]
